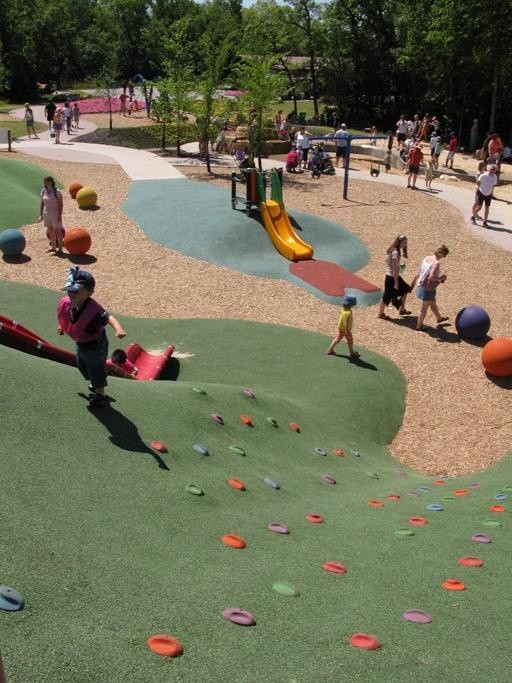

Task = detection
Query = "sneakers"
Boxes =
[470,214,489,229]
[88,383,105,407]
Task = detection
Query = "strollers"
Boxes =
[308,144,335,179]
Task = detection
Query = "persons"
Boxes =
[55,263,128,409]
[409,243,450,333]
[37,174,63,255]
[324,292,362,358]
[384,112,458,190]
[21,96,79,143]
[117,80,163,123]
[371,126,377,145]
[198,114,207,159]
[376,233,412,320]
[469,119,510,226]
[104,348,139,379]
[275,110,349,172]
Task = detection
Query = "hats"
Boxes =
[340,123,348,128]
[342,293,358,306]
[59,268,95,293]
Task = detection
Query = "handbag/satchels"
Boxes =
[419,268,441,290]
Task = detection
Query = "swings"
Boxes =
[370,136,386,177]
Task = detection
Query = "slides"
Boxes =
[0,314,174,381]
[255,167,313,261]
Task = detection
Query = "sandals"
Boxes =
[437,317,449,322]
[379,313,390,319]
[45,249,65,256]
[398,309,411,315]
[416,325,427,330]
